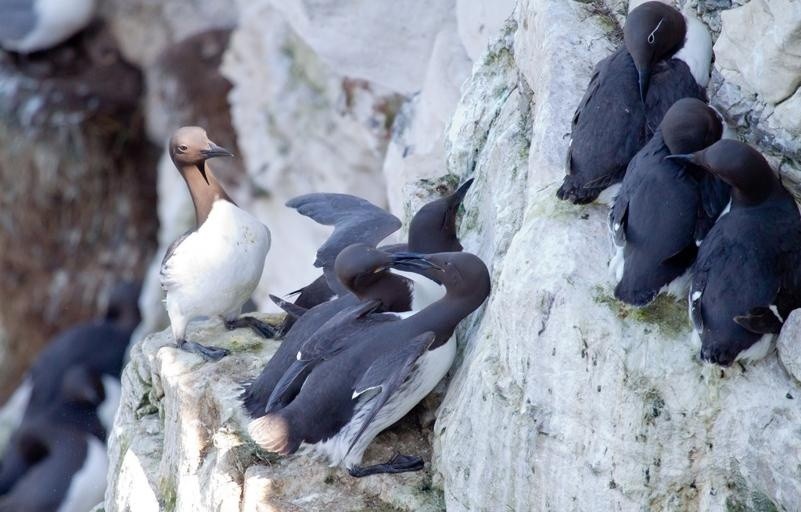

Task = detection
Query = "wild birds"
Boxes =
[243,177,491,481]
[159,125,277,360]
[1,277,144,511]
[1,0,104,72]
[556,0,800,370]
